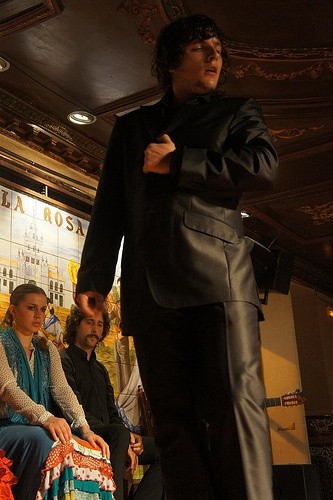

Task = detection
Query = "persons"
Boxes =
[0,284,115,500]
[58,303,165,500]
[72,12,280,500]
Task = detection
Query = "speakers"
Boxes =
[270,464,321,500]
[258,249,295,295]
